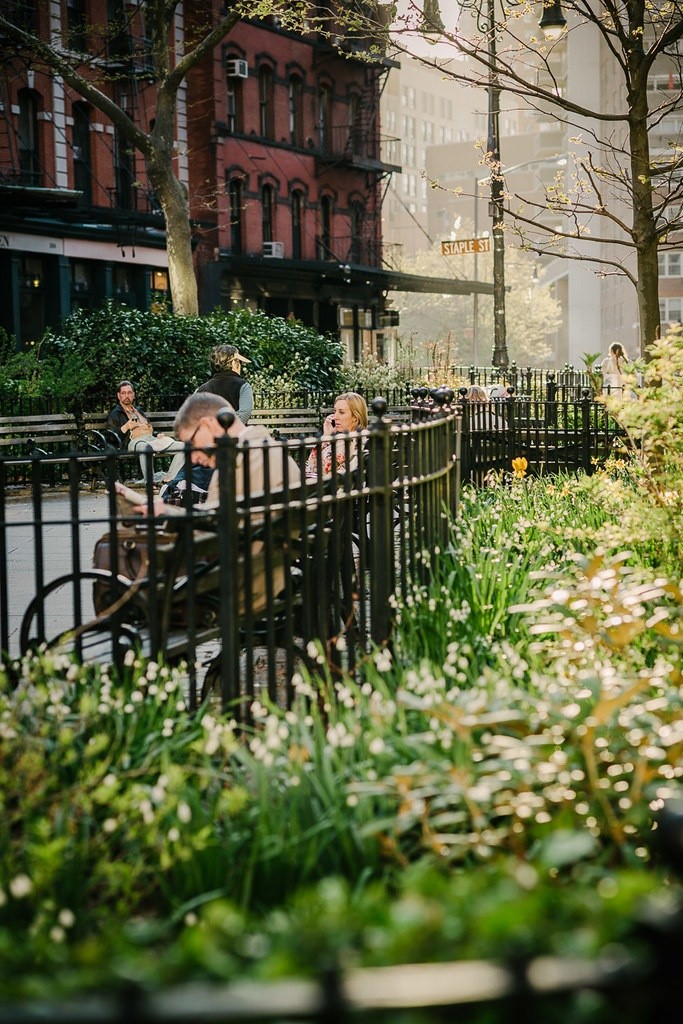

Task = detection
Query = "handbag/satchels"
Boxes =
[93,530,187,583]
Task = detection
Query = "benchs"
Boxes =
[0,413,80,488]
[320,406,412,434]
[246,408,319,463]
[0,468,367,724]
[470,425,638,475]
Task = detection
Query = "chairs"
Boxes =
[77,412,179,490]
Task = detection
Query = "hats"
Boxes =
[211,344,251,363]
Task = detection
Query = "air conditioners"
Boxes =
[227,59,248,78]
[263,241,285,259]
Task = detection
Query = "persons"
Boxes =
[91,391,304,619]
[302,393,368,482]
[600,340,637,401]
[464,384,508,431]
[106,381,185,490]
[193,342,253,425]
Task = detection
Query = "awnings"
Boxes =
[235,250,511,296]
